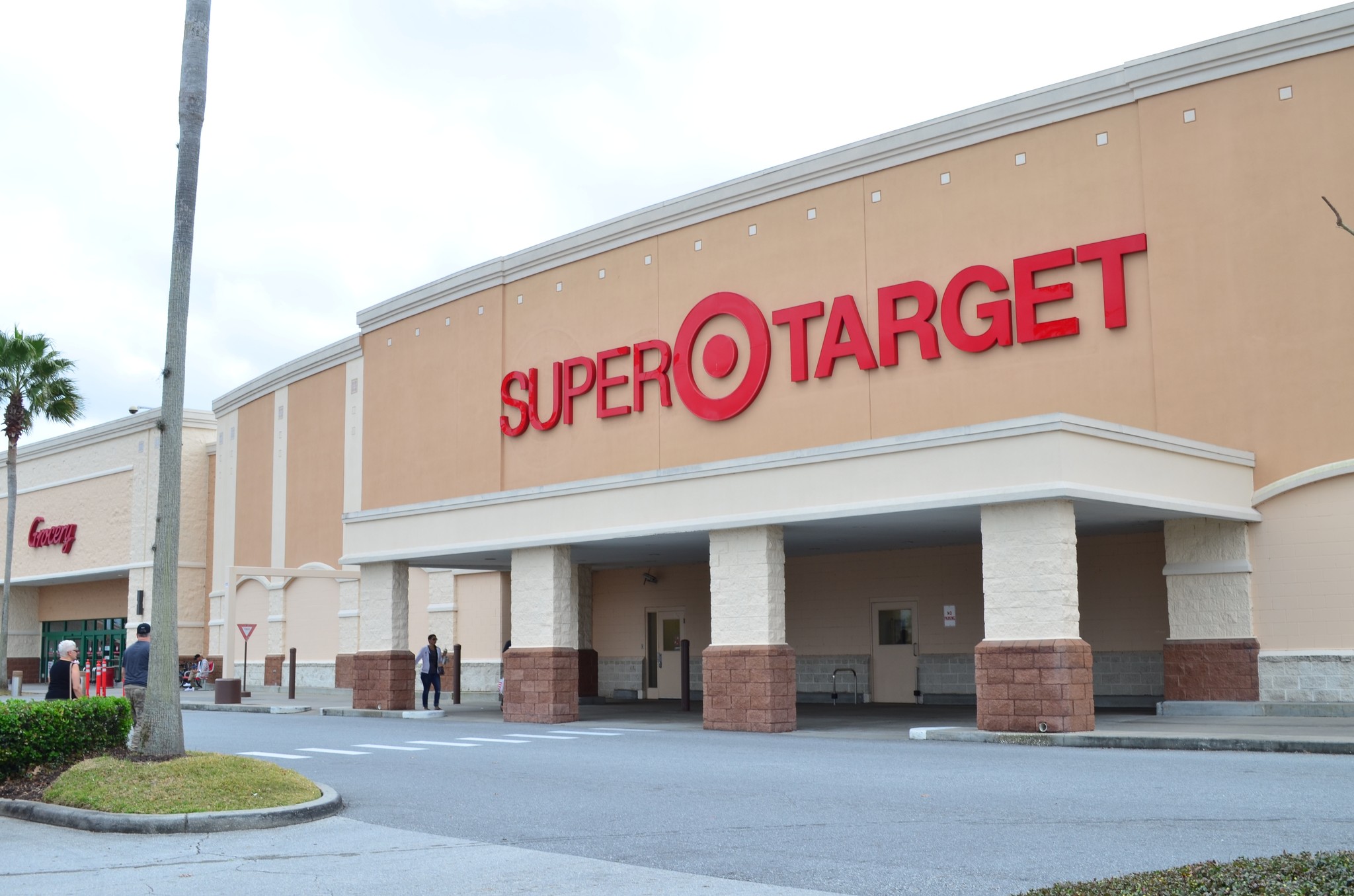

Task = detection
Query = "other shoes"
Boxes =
[435,708,442,710]
[424,708,430,710]
[501,707,503,711]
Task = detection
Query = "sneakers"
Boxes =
[184,687,192,691]
[183,682,191,686]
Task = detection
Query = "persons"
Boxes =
[183,654,209,691]
[415,634,447,711]
[45,640,83,703]
[499,640,512,711]
[121,623,150,752]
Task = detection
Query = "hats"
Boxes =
[137,624,151,634]
[194,654,200,660]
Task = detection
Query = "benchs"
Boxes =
[182,661,215,692]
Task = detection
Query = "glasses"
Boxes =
[430,638,437,640]
[67,650,77,653]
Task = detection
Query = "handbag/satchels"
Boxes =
[498,676,504,695]
[438,666,445,676]
